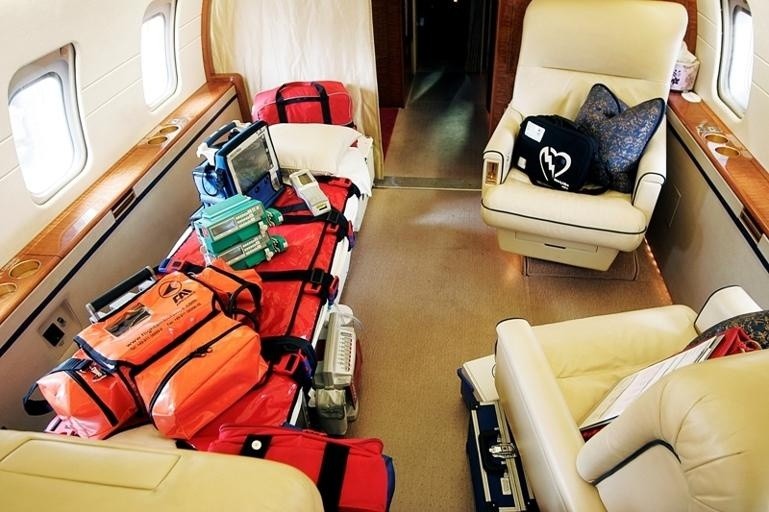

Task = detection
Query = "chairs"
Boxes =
[481,1,689,281]
[458,288,769,512]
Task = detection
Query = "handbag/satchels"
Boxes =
[511,115,613,195]
[73,266,269,443]
[203,423,397,512]
[250,80,357,131]
[22,257,265,439]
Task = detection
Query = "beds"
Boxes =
[48,84,374,460]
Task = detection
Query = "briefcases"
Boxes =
[466,403,539,512]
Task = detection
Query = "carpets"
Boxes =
[378,105,398,161]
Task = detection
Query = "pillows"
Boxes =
[685,308,769,348]
[267,121,360,178]
[576,83,664,192]
